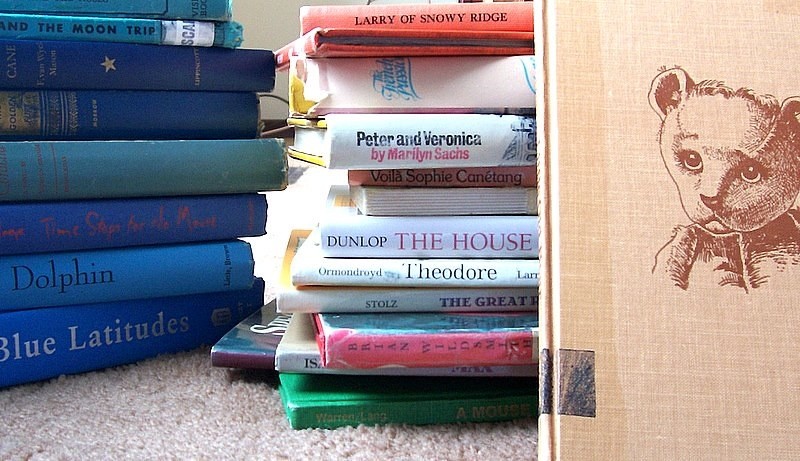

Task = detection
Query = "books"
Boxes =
[273,27,533,71]
[0,0,800,461]
